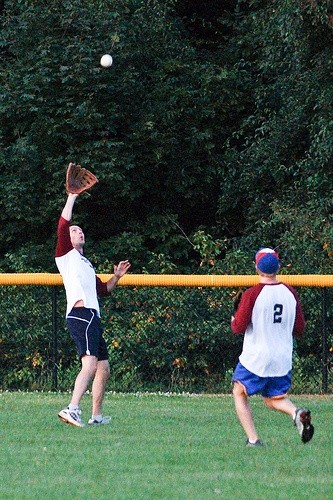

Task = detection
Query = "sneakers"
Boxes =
[244,438,263,447]
[88,416,111,425]
[58,407,84,427]
[293,408,314,444]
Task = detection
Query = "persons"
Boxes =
[54,190,131,428]
[229,248,315,447]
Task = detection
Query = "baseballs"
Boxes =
[100,54,113,67]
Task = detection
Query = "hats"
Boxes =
[255,247,279,276]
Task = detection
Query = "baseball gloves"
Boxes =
[66,163,98,194]
